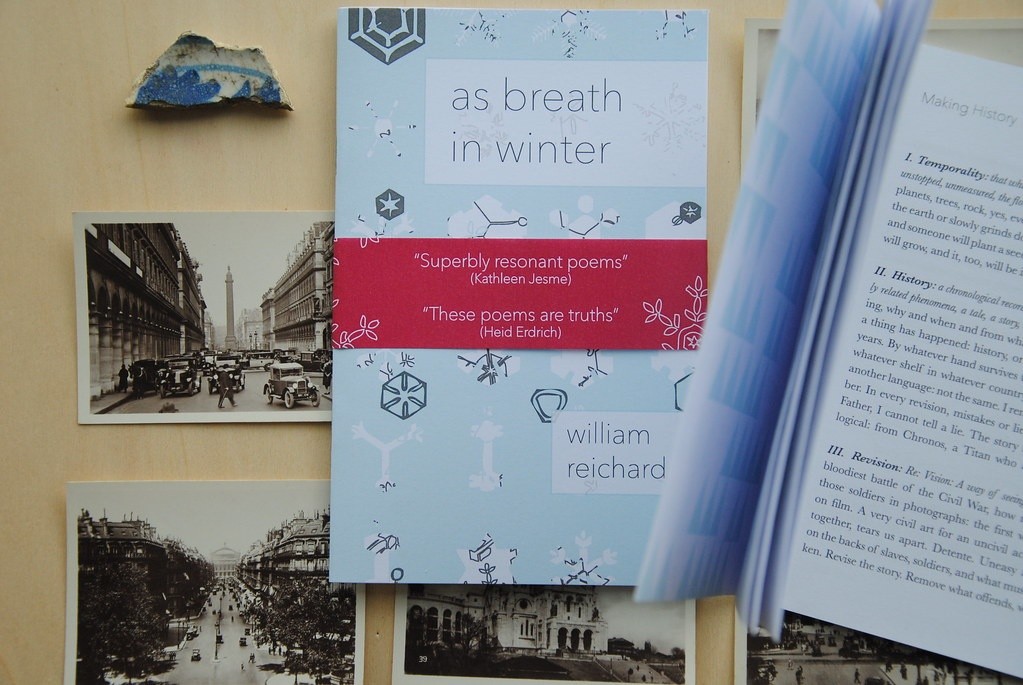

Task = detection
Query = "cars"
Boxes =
[186,581,251,646]
[190,648,201,661]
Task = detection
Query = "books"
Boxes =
[329,7,708,584]
[634,0,1023,685]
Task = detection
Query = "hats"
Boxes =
[223,364,229,368]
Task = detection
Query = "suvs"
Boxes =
[130,348,334,398]
[262,363,324,409]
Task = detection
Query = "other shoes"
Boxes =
[219,406,225,408]
[233,404,238,407]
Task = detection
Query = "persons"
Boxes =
[169,578,282,671]
[771,636,1002,685]
[621,652,653,682]
[118,349,333,408]
